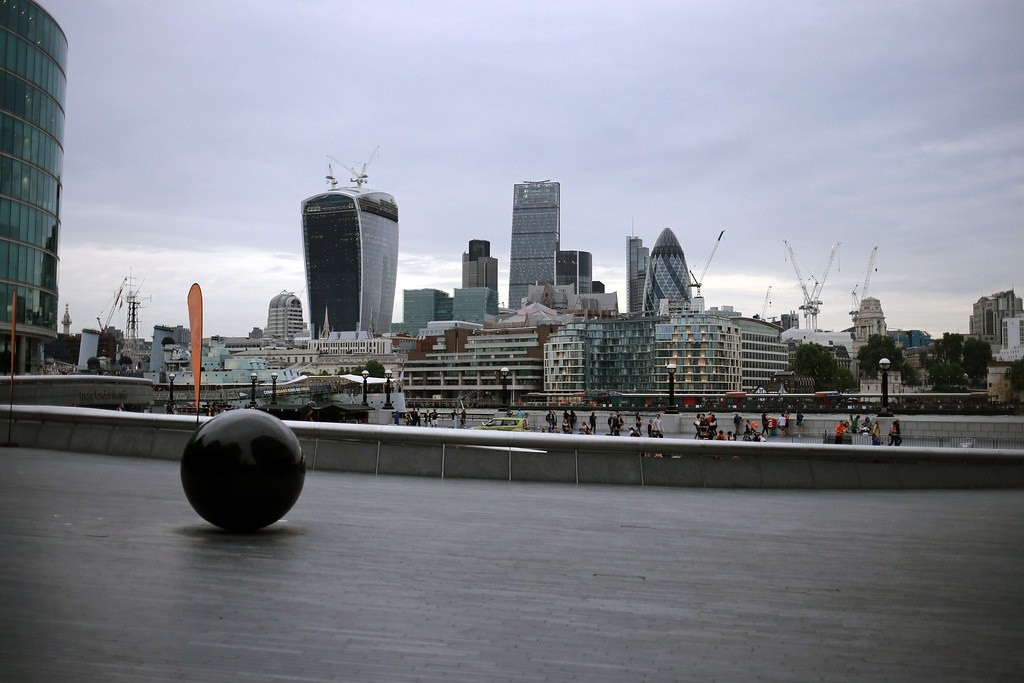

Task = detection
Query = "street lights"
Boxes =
[665,362,680,413]
[167,374,176,405]
[383,369,395,409]
[879,357,895,417]
[271,371,280,404]
[250,372,258,405]
[360,368,370,405]
[500,366,510,412]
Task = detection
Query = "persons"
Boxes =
[648,414,664,438]
[742,420,766,442]
[392,407,466,428]
[843,414,881,445]
[834,420,846,444]
[716,430,726,440]
[728,431,736,441]
[734,413,742,435]
[888,420,902,446]
[506,408,528,418]
[761,412,770,437]
[608,412,624,436]
[166,403,178,414]
[629,426,640,437]
[693,412,717,440]
[546,409,591,435]
[796,409,804,437]
[589,412,597,433]
[777,409,789,437]
[636,412,643,435]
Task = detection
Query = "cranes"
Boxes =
[852,291,863,313]
[781,239,821,317]
[808,240,843,305]
[94,275,128,332]
[688,229,726,298]
[323,145,382,191]
[759,286,774,322]
[858,244,880,300]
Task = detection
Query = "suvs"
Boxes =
[466,417,528,433]
